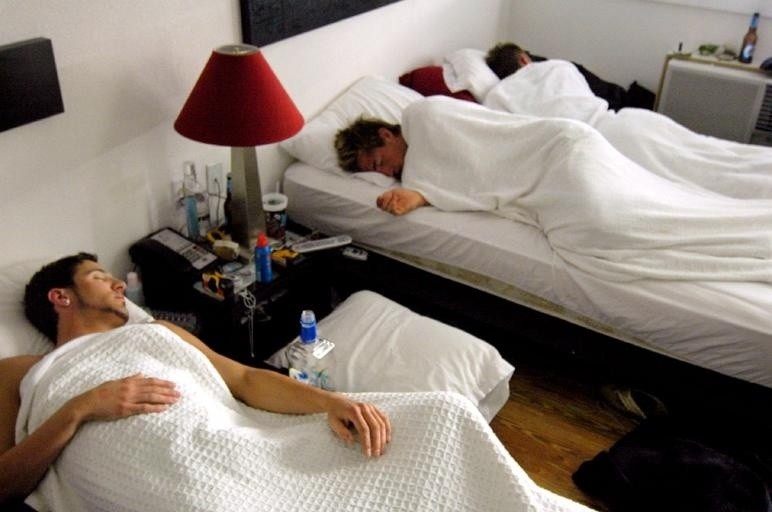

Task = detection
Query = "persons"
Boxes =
[482,42,772,199]
[0,253,594,512]
[335,94,772,284]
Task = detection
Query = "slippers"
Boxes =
[604,381,667,422]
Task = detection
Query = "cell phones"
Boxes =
[340,247,369,261]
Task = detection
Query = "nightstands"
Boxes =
[141,221,335,365]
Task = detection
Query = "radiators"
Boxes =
[754,73,772,141]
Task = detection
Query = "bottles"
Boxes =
[223,173,232,226]
[284,310,337,392]
[254,232,272,282]
[738,11,760,64]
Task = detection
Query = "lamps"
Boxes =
[173,44,305,247]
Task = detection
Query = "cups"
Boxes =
[261,193,286,244]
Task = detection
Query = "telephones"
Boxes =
[128,226,221,290]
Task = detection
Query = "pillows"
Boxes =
[441,42,500,104]
[0,253,154,362]
[280,75,424,188]
[265,290,515,441]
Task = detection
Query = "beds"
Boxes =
[283,160,772,386]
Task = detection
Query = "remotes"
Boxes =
[290,234,353,253]
